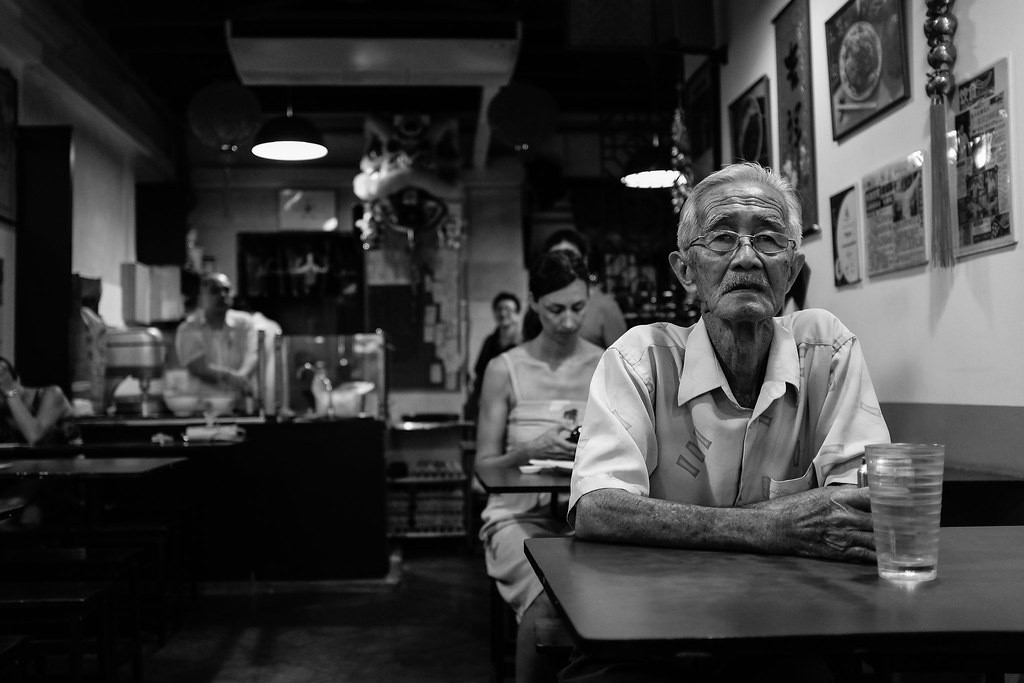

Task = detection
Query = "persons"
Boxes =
[176,272,260,414]
[569,160,896,568]
[0,355,99,609]
[473,233,628,409]
[473,248,608,683]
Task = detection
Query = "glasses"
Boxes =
[683,230,798,256]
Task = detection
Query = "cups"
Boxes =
[864,441,946,583]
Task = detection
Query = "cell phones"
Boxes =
[565,425,583,444]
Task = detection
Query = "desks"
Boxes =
[0,439,231,454]
[74,415,266,440]
[523,523,1024,683]
[472,465,1024,510]
[0,457,189,481]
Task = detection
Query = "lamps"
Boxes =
[251,116,328,162]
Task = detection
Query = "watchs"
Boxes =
[6,388,18,398]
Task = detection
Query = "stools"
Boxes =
[0,521,168,683]
